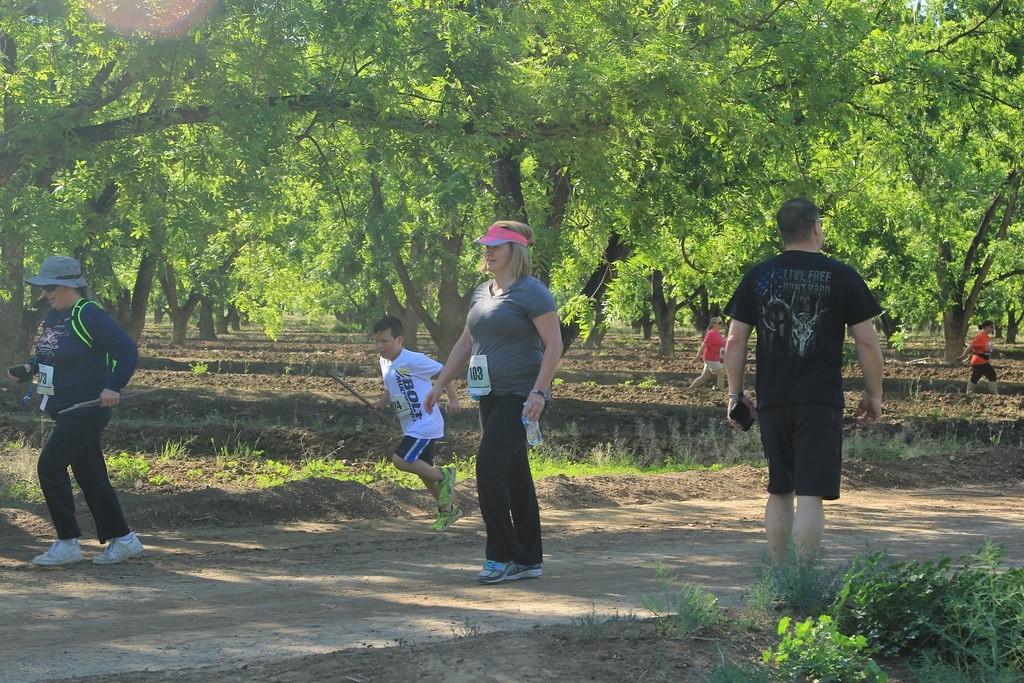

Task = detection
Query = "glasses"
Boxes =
[39,285,56,292]
[717,322,723,325]
[990,325,995,330]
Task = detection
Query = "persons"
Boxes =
[689,317,727,390]
[958,319,999,395]
[8,255,145,565]
[422,221,564,583]
[721,198,884,567]
[367,316,464,532]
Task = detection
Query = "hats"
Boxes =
[473,226,528,247]
[24,256,88,289]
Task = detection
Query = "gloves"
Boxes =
[7,360,39,382]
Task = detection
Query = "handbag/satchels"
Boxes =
[971,351,991,360]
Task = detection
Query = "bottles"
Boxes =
[521,402,543,447]
[988,340,994,354]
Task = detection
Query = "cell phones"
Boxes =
[9,364,31,384]
[730,400,755,432]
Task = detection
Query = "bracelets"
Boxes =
[533,390,546,399]
[728,392,743,398]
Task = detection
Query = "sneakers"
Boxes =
[33,538,83,565]
[92,532,144,563]
[428,503,463,531]
[477,558,518,584]
[503,560,543,580]
[437,465,457,506]
